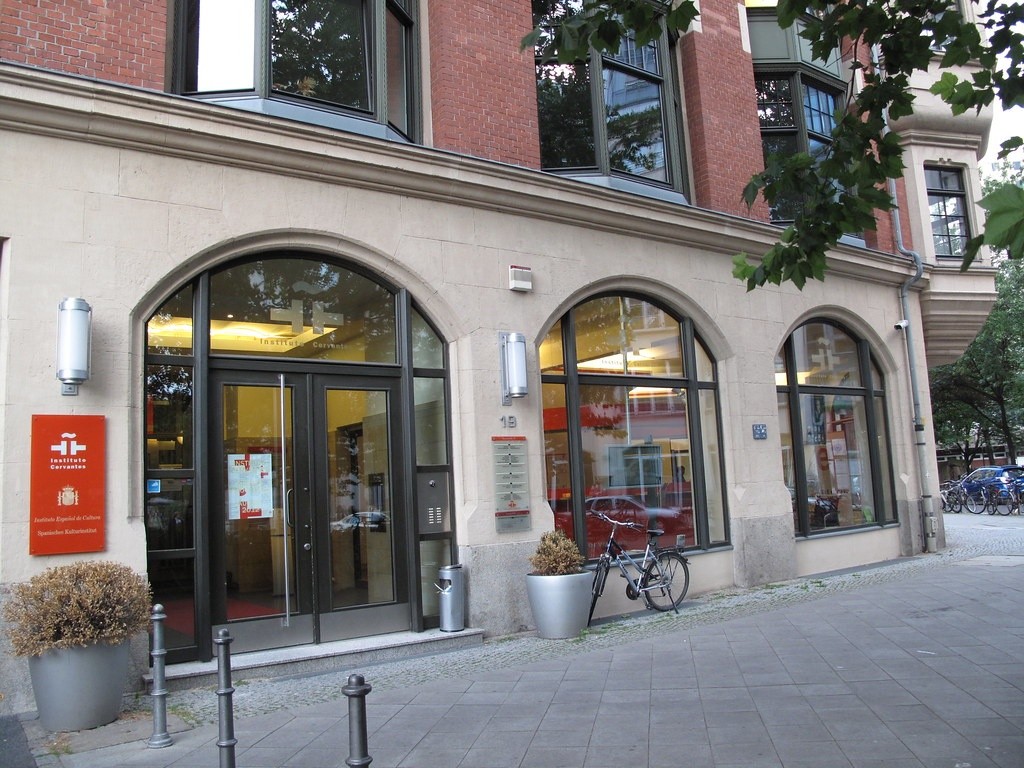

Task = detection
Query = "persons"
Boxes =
[676,466,686,482]
[810,400,824,442]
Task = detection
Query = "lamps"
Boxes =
[55,296,95,398]
[498,330,529,407]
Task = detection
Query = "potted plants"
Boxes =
[1,560,155,733]
[524,528,594,640]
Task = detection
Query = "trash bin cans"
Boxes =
[433,563,466,632]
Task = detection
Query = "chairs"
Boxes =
[809,495,840,530]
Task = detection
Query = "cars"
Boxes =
[330,512,391,535]
[787,487,868,527]
[554,484,698,559]
[952,464,1024,504]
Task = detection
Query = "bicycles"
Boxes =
[938,477,1024,516]
[585,508,690,628]
